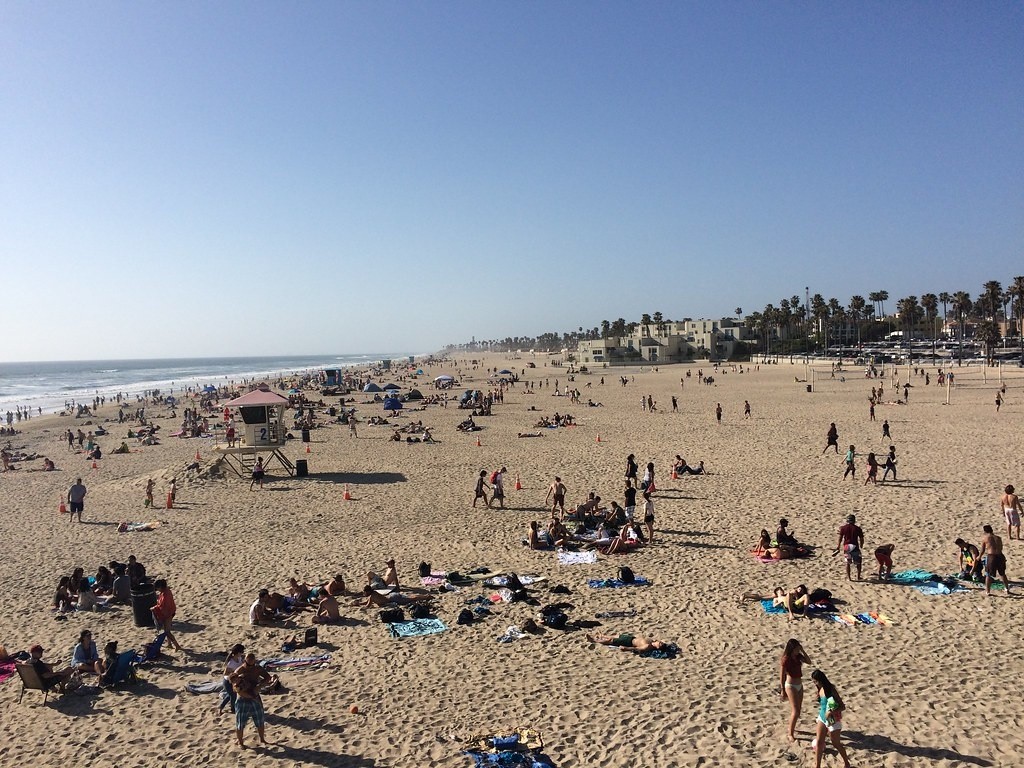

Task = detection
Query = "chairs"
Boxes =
[15,663,65,706]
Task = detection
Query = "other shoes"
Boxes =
[497,635,512,643]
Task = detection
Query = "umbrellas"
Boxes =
[289,389,303,393]
[387,390,399,397]
[204,387,216,391]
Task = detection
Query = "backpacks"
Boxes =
[418,561,431,577]
[617,567,635,584]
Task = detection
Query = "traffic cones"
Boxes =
[196,449,199,459]
[305,444,309,455]
[343,483,351,499]
[165,491,173,509]
[58,496,66,513]
[92,458,96,469]
[596,433,602,444]
[515,474,522,490]
[476,434,483,446]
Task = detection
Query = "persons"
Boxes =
[0,353,1024,768]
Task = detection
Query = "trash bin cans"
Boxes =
[130,583,157,625]
[295,459,308,477]
[347,389,350,394]
[329,407,336,416]
[302,430,310,442]
[339,398,345,406]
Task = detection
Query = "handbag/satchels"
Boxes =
[379,608,404,623]
[409,604,430,619]
[540,605,568,629]
[457,609,473,625]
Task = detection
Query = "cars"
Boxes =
[526,362,536,368]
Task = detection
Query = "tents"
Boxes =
[416,368,424,374]
[384,383,401,389]
[499,369,512,374]
[435,374,454,389]
[363,383,382,392]
[383,398,403,410]
[409,389,423,399]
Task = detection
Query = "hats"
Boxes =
[333,575,344,581]
[30,644,45,653]
[81,630,92,637]
[385,559,395,565]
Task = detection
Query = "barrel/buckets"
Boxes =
[130,583,156,627]
[296,459,308,476]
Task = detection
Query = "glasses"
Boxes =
[246,661,256,669]
